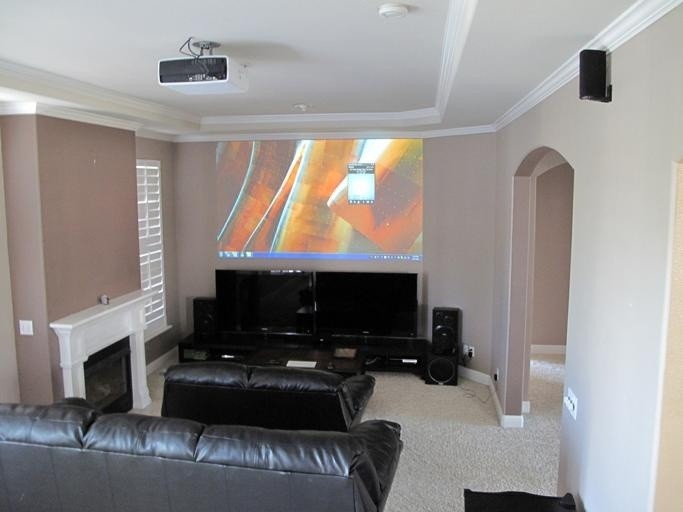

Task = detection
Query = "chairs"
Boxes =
[464,488,578,512]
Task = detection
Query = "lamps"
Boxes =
[379,3,409,20]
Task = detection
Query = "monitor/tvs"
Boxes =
[315,272,418,351]
[214,269,313,348]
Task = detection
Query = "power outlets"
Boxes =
[468,346,475,357]
[491,367,499,385]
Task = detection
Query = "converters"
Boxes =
[469,349,472,357]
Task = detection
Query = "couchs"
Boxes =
[0,405,405,512]
[160,361,375,431]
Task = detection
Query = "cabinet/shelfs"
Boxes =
[178,335,430,382]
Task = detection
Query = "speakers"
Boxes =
[157,55,228,86]
[432,307,461,355]
[425,352,459,385]
[579,50,605,98]
[193,297,218,337]
